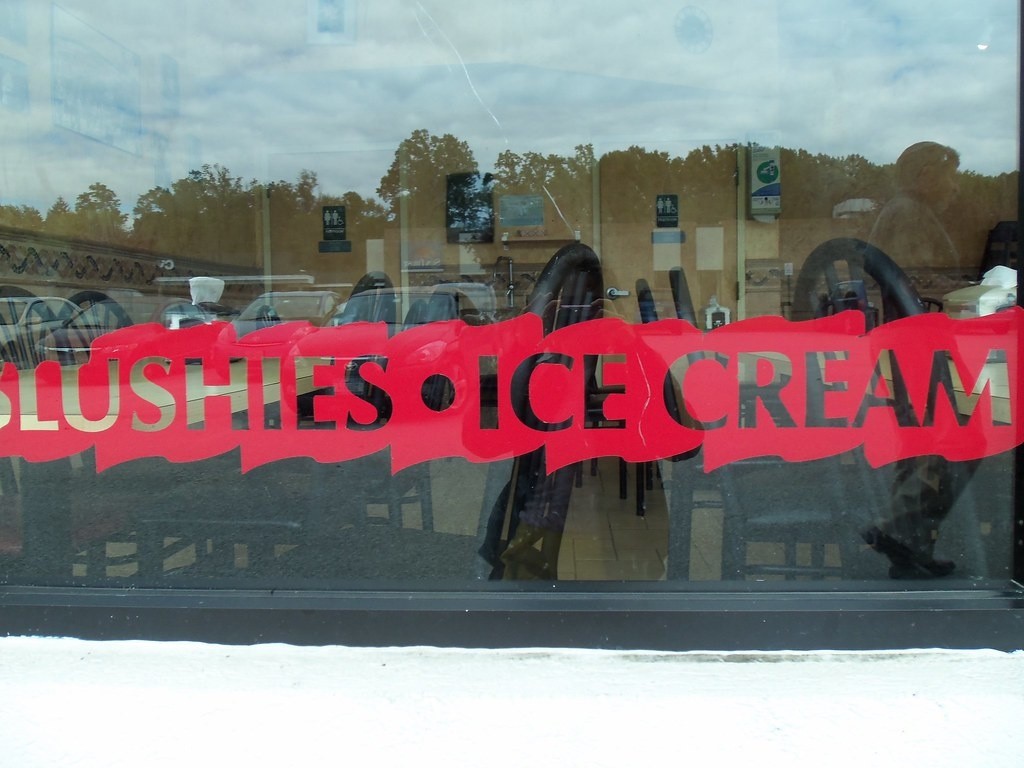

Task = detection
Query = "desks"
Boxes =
[0,357,335,588]
[736,339,1024,592]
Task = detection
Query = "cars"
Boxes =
[0,296,85,364]
[212,291,346,364]
[33,296,214,366]
[285,286,507,418]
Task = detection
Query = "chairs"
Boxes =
[0,238,1024,594]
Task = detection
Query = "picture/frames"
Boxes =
[308,0,355,43]
